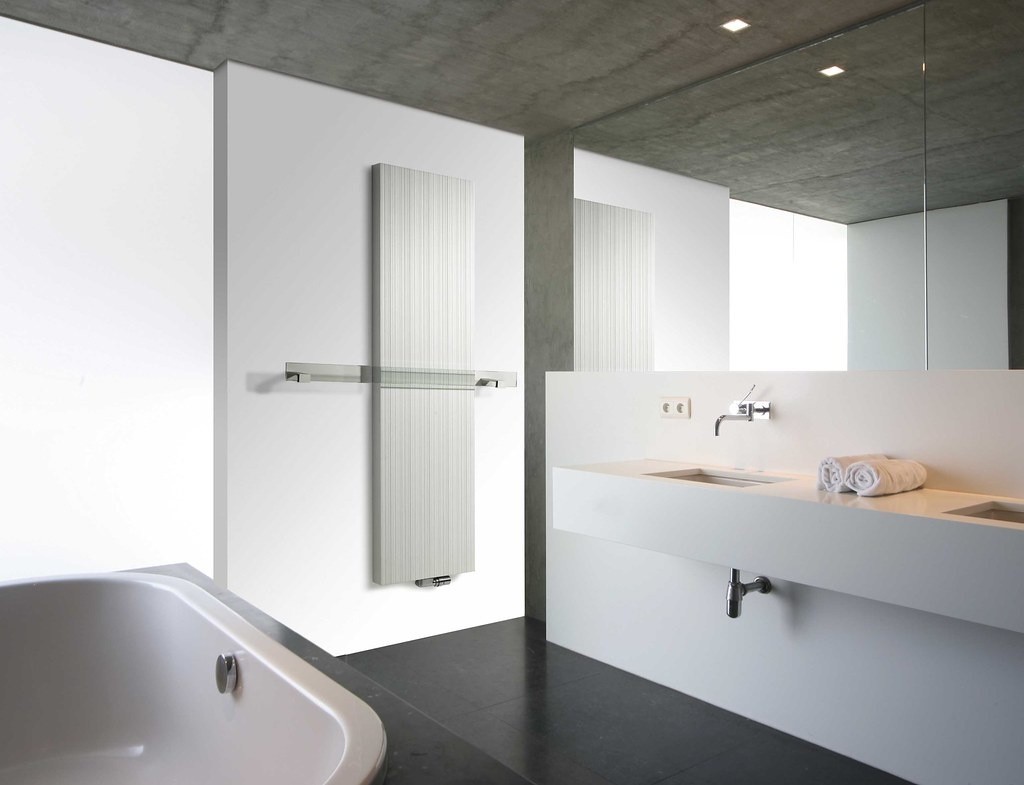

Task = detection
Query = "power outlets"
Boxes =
[658,397,691,419]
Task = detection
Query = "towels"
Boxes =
[816,453,928,498]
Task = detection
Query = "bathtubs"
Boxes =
[1,569,389,785]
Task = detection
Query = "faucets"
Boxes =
[712,384,771,437]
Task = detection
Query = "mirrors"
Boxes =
[573,0,1024,374]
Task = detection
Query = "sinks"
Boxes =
[941,499,1024,524]
[640,467,800,489]
